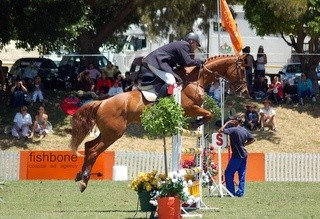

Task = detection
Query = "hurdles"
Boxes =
[170,88,222,218]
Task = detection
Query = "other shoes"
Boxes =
[25,136,29,141]
[16,137,21,141]
[28,137,33,141]
[35,139,40,143]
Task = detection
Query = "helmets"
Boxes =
[186,33,201,47]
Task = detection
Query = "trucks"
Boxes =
[115,6,313,76]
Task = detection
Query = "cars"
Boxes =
[7,58,77,98]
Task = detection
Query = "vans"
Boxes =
[100,35,167,77]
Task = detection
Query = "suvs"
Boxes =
[57,53,122,89]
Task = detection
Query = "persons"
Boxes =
[218,116,256,196]
[0,61,53,144]
[211,46,320,132]
[76,62,137,108]
[138,32,206,101]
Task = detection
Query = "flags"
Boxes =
[219,0,244,57]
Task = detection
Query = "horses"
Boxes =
[68,54,248,193]
